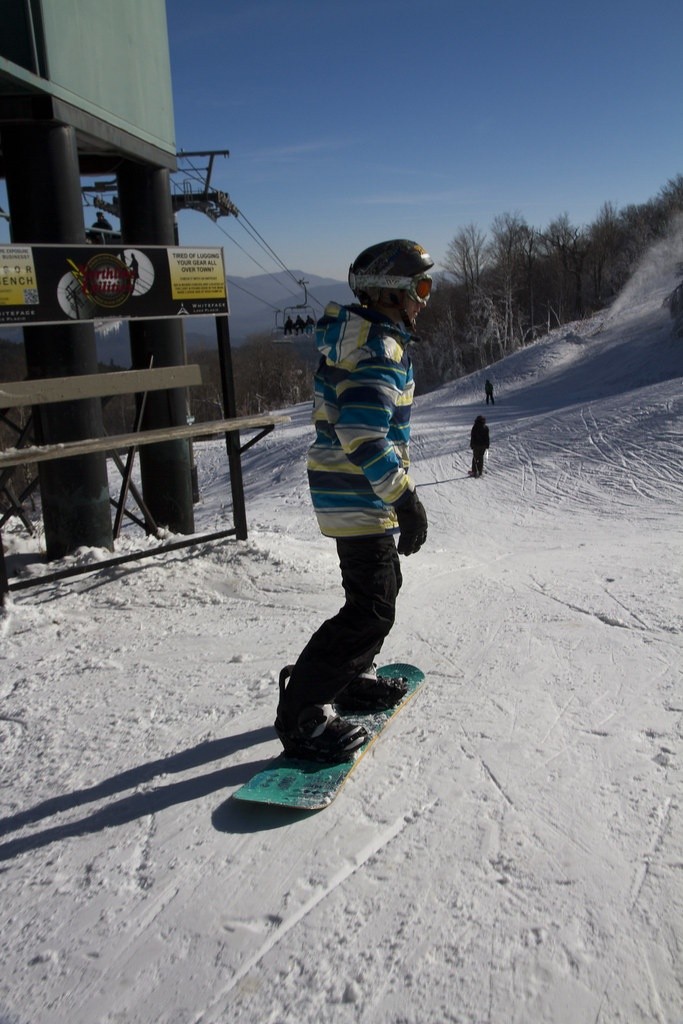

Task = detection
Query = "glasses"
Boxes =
[406,274,434,307]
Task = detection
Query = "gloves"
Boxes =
[394,486,428,556]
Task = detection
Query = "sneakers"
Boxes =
[275,665,369,759]
[336,664,407,710]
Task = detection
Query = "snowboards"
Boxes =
[231,663,424,810]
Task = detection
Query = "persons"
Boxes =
[468,416,490,478]
[87,211,113,244]
[272,240,436,760]
[484,378,495,405]
[282,314,314,335]
[128,253,139,294]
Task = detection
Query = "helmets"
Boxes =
[350,239,435,305]
